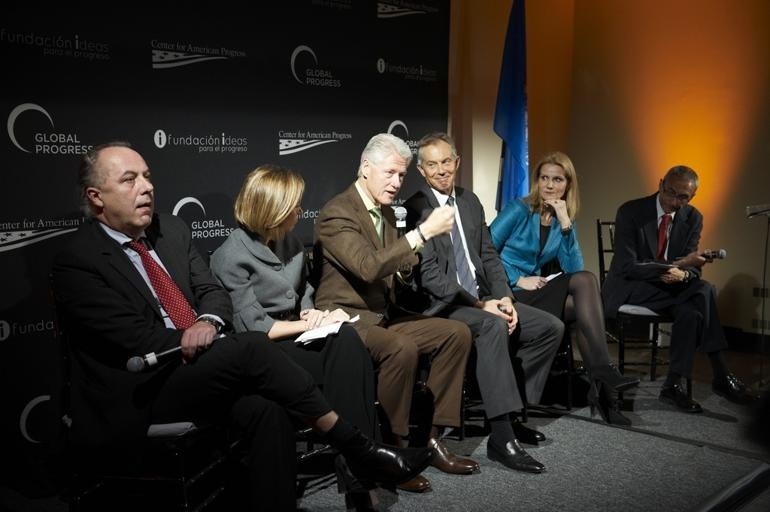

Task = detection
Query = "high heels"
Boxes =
[588,362,640,426]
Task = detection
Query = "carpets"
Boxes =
[289,371,770,511]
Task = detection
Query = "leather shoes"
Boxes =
[659,384,701,413]
[712,371,753,405]
[512,420,546,441]
[337,437,480,510]
[487,436,545,473]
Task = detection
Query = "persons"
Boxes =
[48,142,438,512]
[308,131,481,494]
[486,148,640,428]
[207,162,375,510]
[602,164,761,414]
[397,131,565,474]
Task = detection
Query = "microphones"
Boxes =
[394,207,408,240]
[701,248,727,261]
[127,346,181,372]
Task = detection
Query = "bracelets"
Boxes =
[683,271,689,282]
[416,225,427,243]
[196,316,221,334]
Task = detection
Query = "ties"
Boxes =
[447,196,479,301]
[369,207,384,246]
[657,213,672,261]
[127,240,195,330]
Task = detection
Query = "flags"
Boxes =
[491,0,531,215]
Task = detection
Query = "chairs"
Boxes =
[46,219,693,512]
